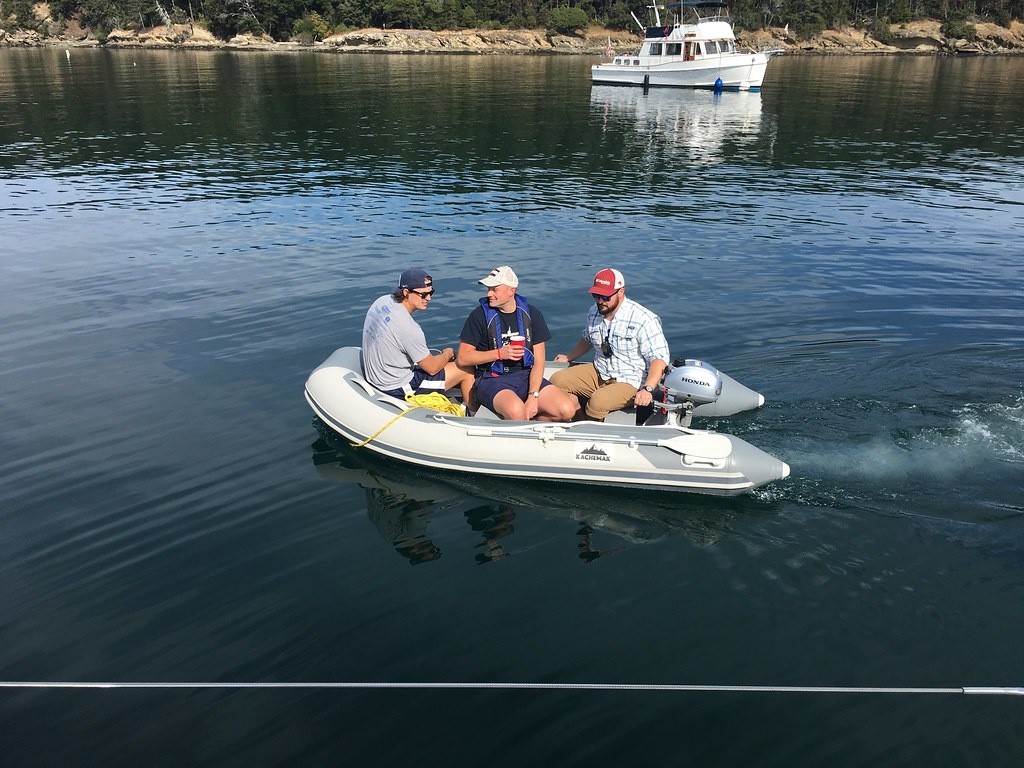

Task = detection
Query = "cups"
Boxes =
[509,336,525,360]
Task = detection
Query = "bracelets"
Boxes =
[498,349,501,360]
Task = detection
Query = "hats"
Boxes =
[477,265,519,288]
[398,267,433,290]
[587,267,625,297]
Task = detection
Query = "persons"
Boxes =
[457,266,576,422]
[361,267,480,417]
[549,269,670,421]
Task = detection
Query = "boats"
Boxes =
[303,345,793,498]
[589,79,763,162]
[591,0,770,92]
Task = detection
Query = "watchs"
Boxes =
[528,392,539,397]
[642,385,653,392]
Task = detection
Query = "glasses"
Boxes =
[407,288,435,299]
[592,288,620,302]
